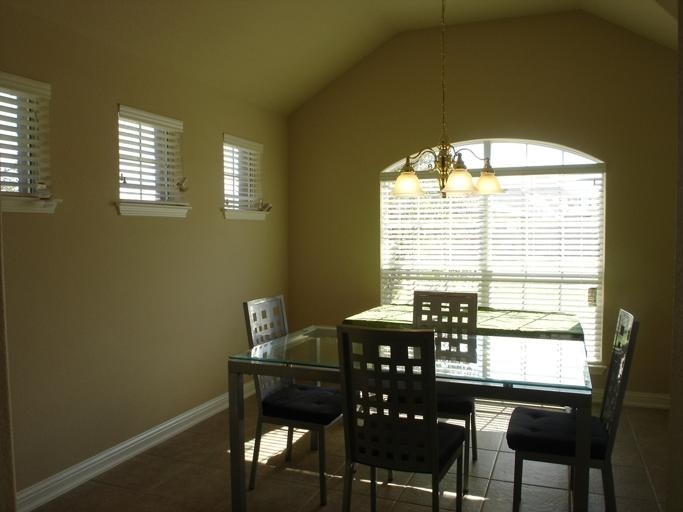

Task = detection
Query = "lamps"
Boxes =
[392,1,504,199]
